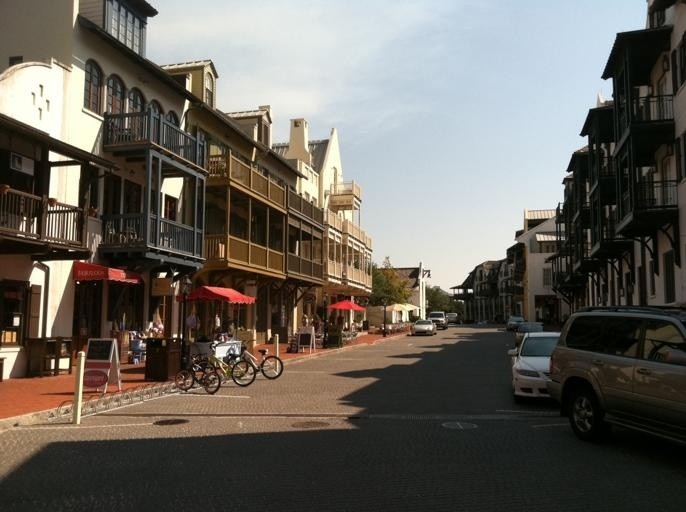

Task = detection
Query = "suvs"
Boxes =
[427,311,449,330]
[543,303,686,456]
[445,312,459,324]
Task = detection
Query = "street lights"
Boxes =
[322,291,328,349]
[177,272,193,376]
[382,298,388,337]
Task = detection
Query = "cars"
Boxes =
[410,320,438,336]
[513,322,544,348]
[506,314,527,331]
[507,332,562,403]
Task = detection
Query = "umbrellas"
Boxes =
[381,303,419,323]
[327,300,365,328]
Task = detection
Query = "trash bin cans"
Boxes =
[363,320,369,330]
[145,338,182,381]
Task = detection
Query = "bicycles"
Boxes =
[227,338,284,380]
[190,340,257,388]
[174,352,222,396]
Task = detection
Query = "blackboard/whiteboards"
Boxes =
[298,334,312,345]
[84,338,115,362]
